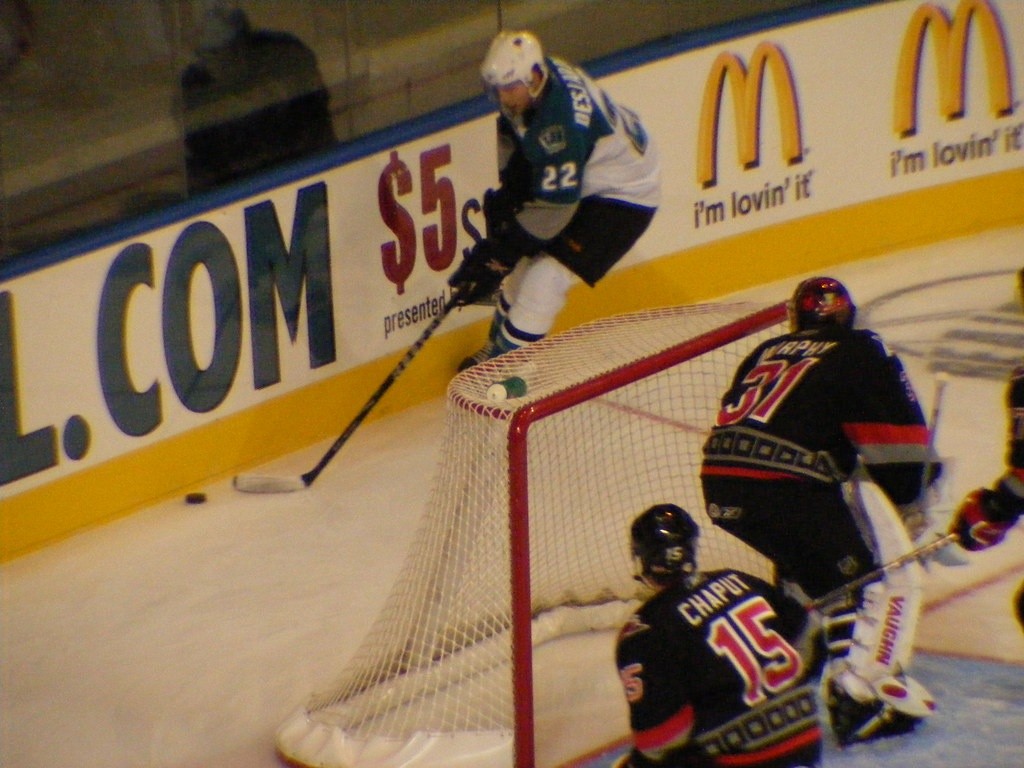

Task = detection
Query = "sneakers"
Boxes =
[458,342,502,377]
[827,680,921,748]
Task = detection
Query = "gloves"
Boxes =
[448,241,512,306]
[949,488,1014,551]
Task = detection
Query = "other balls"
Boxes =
[184,492,207,505]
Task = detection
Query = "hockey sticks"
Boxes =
[231,275,477,495]
[915,373,952,511]
[802,531,962,613]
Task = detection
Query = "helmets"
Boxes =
[630,503,701,586]
[789,277,856,333]
[478,28,548,97]
[194,8,250,77]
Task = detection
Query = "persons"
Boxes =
[700,278,943,745]
[171,7,337,199]
[949,264,1024,631]
[613,505,828,768]
[450,30,659,374]
